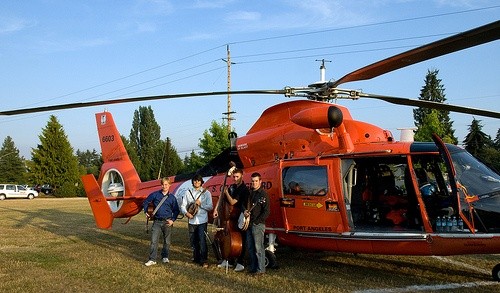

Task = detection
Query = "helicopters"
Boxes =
[0,19,500,271]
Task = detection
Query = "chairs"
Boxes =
[374,164,403,200]
[403,165,455,231]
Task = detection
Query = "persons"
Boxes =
[181,174,214,268]
[213,169,250,272]
[241,171,270,276]
[287,181,301,195]
[144,177,180,266]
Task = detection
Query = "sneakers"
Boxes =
[234,263,244,272]
[145,260,157,267]
[162,257,169,263]
[217,261,233,267]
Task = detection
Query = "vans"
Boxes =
[0,183,15,192]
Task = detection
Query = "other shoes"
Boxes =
[252,271,265,275]
[203,263,209,268]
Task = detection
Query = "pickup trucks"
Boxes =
[0,185,38,200]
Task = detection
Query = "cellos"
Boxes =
[212,161,243,275]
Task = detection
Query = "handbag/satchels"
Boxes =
[238,213,251,230]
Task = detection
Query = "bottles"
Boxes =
[221,260,228,275]
[452,216,457,233]
[436,216,441,233]
[447,216,452,233]
[442,216,446,233]
[458,216,463,233]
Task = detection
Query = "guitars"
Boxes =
[237,194,266,230]
[187,186,210,216]
[148,202,155,221]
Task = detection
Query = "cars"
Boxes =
[23,185,53,195]
